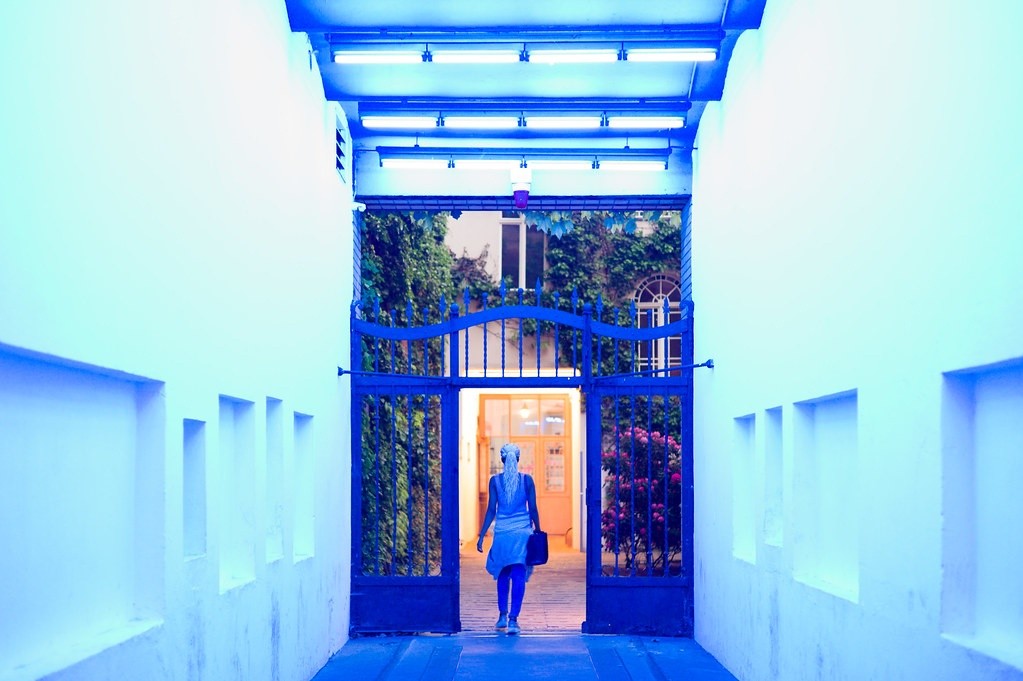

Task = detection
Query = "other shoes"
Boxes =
[508,620,520,632]
[496,616,507,628]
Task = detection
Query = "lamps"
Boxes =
[328,30,728,64]
[374,146,674,169]
[361,113,689,130]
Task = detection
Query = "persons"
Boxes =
[477,444,541,634]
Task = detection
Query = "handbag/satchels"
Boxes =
[526,531,548,565]
